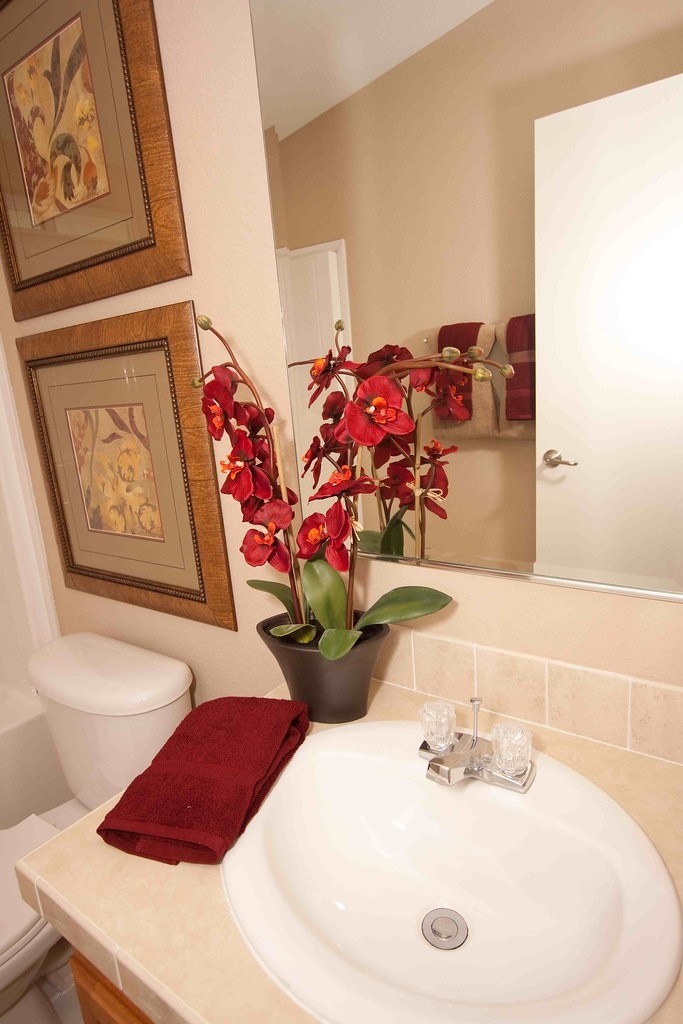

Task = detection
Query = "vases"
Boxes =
[256,608,391,716]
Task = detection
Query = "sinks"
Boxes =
[221,749,682,1024]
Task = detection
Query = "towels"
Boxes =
[96,695,310,866]
[433,314,536,441]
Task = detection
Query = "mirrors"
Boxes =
[245,2,682,604]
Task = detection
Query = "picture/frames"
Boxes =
[16,301,239,632]
[0,0,192,323]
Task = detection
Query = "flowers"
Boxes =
[289,319,517,558]
[192,305,492,644]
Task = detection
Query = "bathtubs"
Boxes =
[0,685,75,830]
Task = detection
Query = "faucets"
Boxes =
[418,697,537,794]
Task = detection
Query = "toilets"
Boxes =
[0,631,195,1024]
